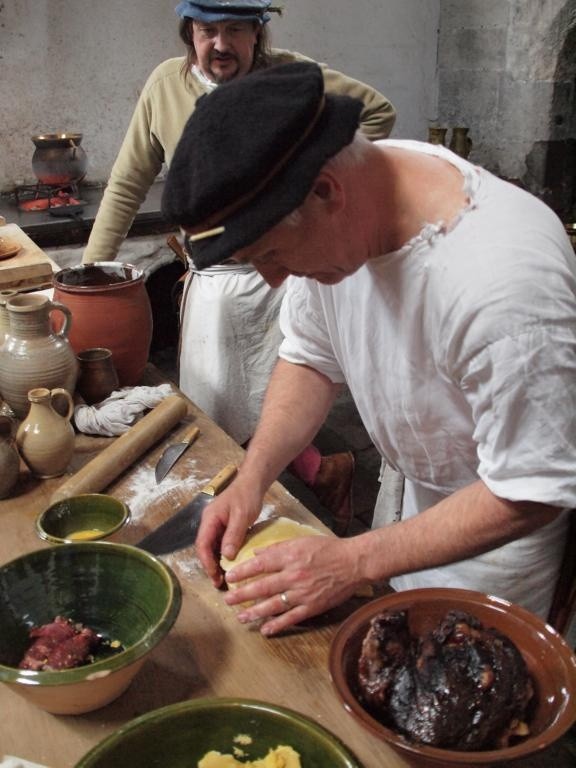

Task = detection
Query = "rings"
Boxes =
[280,592,292,610]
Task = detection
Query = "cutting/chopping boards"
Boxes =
[0,223,53,284]
[0,379,449,768]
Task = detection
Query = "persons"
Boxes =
[79,0,396,539]
[161,62,576,637]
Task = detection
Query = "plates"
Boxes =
[0,243,21,258]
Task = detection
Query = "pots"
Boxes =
[31,132,89,186]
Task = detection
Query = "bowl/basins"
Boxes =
[73,696,363,768]
[327,588,576,765]
[34,494,133,543]
[0,541,182,714]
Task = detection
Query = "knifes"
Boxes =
[134,463,238,554]
[155,426,200,485]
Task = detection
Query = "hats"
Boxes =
[174,0,272,24]
[158,61,365,271]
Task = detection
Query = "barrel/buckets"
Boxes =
[50,262,154,387]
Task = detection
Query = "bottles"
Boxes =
[0,290,78,419]
[76,348,119,407]
[0,416,21,499]
[16,388,76,479]
[429,127,472,159]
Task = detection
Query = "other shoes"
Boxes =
[313,451,356,538]
